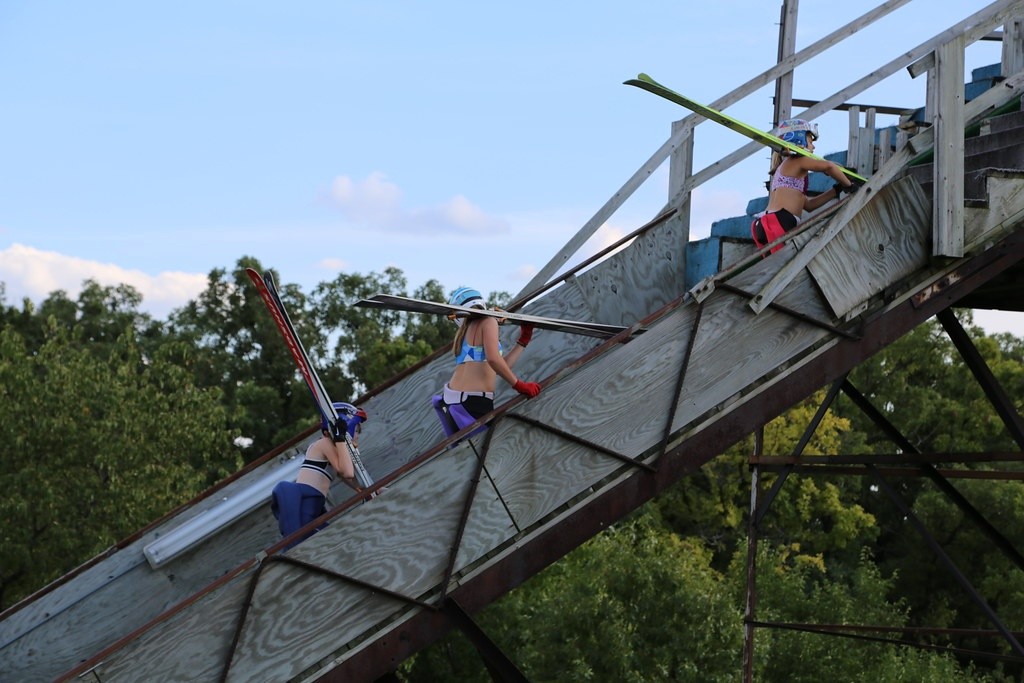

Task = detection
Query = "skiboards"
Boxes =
[621,72,870,186]
[245,265,379,500]
[352,292,649,343]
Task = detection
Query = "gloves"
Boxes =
[516,324,533,347]
[833,167,857,194]
[512,378,540,401]
[328,417,348,442]
[841,183,859,194]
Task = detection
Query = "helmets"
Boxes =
[777,119,818,156]
[450,287,487,328]
[321,403,367,440]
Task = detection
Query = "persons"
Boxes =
[752,118,861,254]
[269,402,367,550]
[440,287,542,433]
